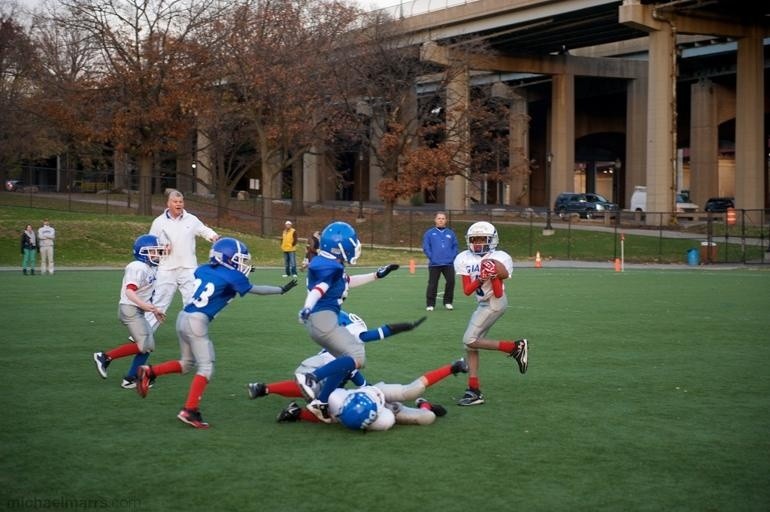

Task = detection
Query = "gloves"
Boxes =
[478,259,498,283]
[375,262,400,278]
[298,308,311,324]
[278,280,298,296]
[386,316,426,335]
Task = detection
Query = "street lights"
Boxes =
[189,159,221,227]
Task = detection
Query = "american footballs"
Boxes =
[480,259,509,279]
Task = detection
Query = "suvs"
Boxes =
[704,197,736,214]
[554,191,622,220]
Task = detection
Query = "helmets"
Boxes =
[465,220,499,257]
[335,384,386,434]
[132,233,169,268]
[318,221,362,267]
[208,237,254,277]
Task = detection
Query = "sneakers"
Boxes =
[457,387,485,406]
[245,381,269,401]
[120,376,156,389]
[445,303,454,310]
[281,273,297,279]
[92,350,109,380]
[414,397,447,418]
[273,401,301,424]
[305,398,332,424]
[22,269,55,277]
[450,356,469,378]
[135,364,154,399]
[295,372,321,404]
[508,338,529,375]
[177,406,210,431]
[426,306,434,311]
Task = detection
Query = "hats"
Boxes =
[285,220,292,225]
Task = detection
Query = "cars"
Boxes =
[4,180,39,193]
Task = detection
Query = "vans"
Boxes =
[630,184,700,221]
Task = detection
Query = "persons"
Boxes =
[128,191,223,344]
[299,231,322,273]
[91,234,169,389]
[453,221,529,406]
[245,310,428,424]
[136,238,299,430]
[37,218,56,276]
[421,211,460,312]
[280,221,298,279]
[297,220,366,425]
[327,356,469,432]
[20,224,38,276]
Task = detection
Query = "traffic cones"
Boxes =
[533,251,544,268]
